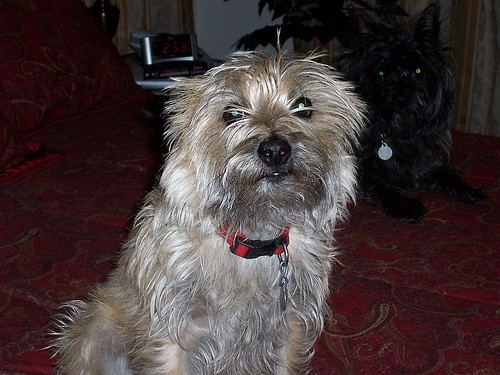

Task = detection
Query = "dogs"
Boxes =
[331,0,487,222]
[40,30,370,375]
